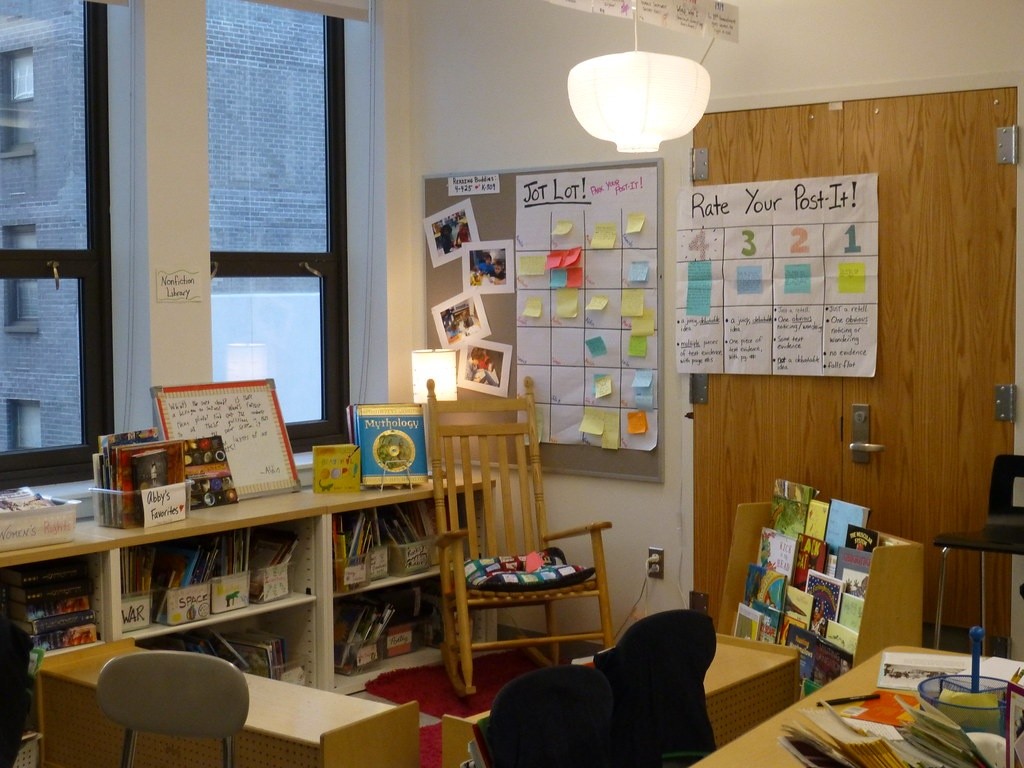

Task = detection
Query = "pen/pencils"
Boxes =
[1011,667,1020,682]
[817,694,880,707]
[1014,669,1024,683]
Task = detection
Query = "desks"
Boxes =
[691,645,1024,768]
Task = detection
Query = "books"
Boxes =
[120,527,298,622]
[185,435,239,510]
[0,486,55,509]
[313,443,361,493]
[0,557,98,652]
[733,479,880,700]
[778,652,1024,768]
[160,626,288,680]
[93,427,185,528]
[334,587,422,674]
[332,504,432,588]
[347,403,429,485]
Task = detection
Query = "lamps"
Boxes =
[567,0,709,153]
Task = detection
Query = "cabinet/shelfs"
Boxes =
[0,468,497,740]
[718,502,924,690]
[37,643,420,768]
[443,631,801,768]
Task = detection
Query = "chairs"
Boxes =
[933,454,1024,648]
[96,650,252,767]
[595,607,716,767]
[484,661,613,768]
[428,378,611,694]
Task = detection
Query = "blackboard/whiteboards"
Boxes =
[151,378,302,507]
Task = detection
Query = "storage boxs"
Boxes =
[204,570,252,614]
[88,479,194,529]
[332,551,372,592]
[248,560,296,605]
[334,639,378,675]
[0,495,82,552]
[390,534,437,577]
[153,580,212,624]
[366,544,390,580]
[119,587,156,632]
[382,621,413,657]
[271,655,306,685]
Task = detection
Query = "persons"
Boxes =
[442,309,473,330]
[432,212,469,254]
[465,347,499,387]
[473,250,506,283]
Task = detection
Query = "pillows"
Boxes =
[448,548,595,593]
